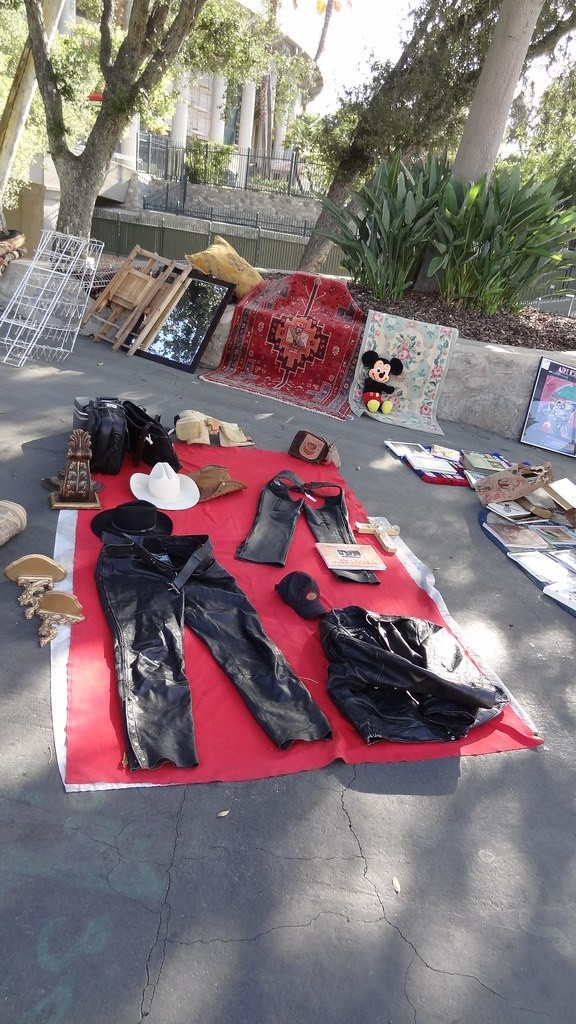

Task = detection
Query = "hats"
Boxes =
[275,570,327,618]
[91,500,172,538]
[188,465,247,502]
[130,462,200,510]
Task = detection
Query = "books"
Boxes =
[385,440,576,612]
[315,542,387,571]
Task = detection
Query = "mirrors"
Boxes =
[121,266,237,374]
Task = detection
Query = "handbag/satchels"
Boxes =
[82,397,181,473]
[473,463,554,505]
[288,430,331,464]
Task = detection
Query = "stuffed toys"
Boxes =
[362,350,403,414]
